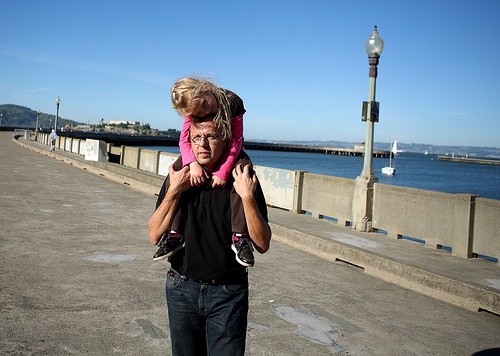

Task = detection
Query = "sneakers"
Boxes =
[230,233,255,267]
[152,231,188,262]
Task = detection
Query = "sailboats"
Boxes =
[382,134,397,175]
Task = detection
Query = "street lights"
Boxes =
[55,96,61,132]
[361,25,384,180]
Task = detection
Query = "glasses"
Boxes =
[189,133,221,145]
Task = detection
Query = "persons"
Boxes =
[50,129,57,151]
[148,115,272,356]
[151,78,255,267]
